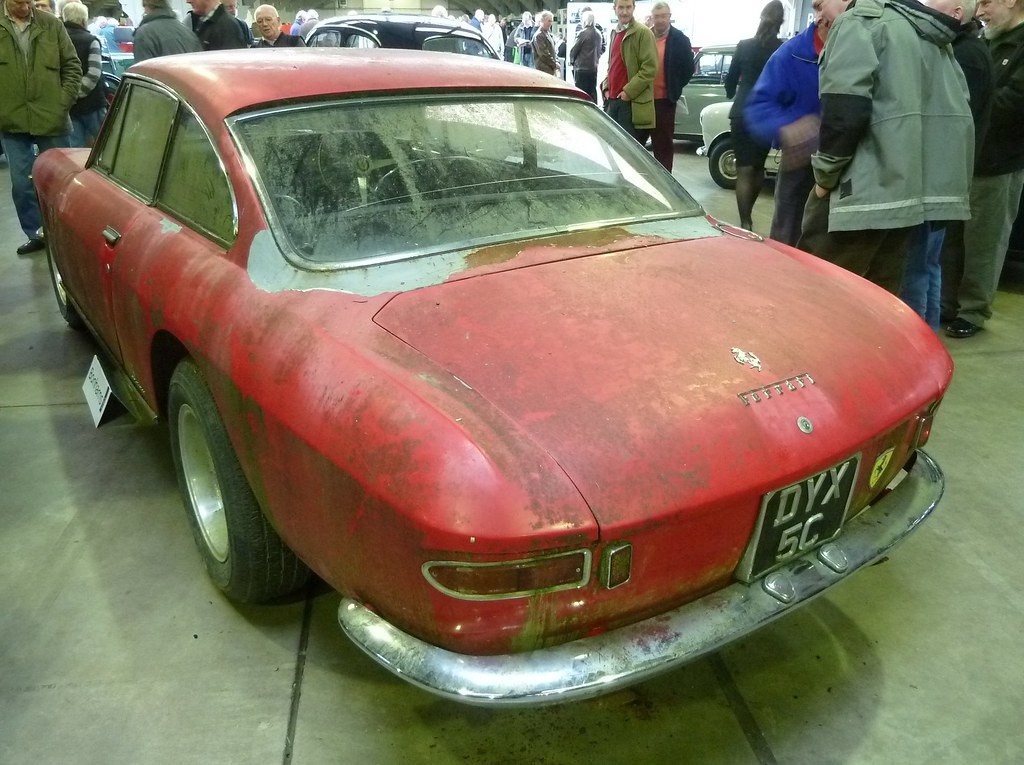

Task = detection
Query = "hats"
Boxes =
[760,0,783,23]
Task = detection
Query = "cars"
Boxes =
[297,11,503,62]
[673,46,742,145]
[696,93,784,190]
[32,45,955,713]
[95,25,137,117]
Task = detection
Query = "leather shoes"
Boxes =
[17,239,45,254]
[940,315,984,337]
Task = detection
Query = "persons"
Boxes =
[431,0,1024,337]
[0,0,365,256]
